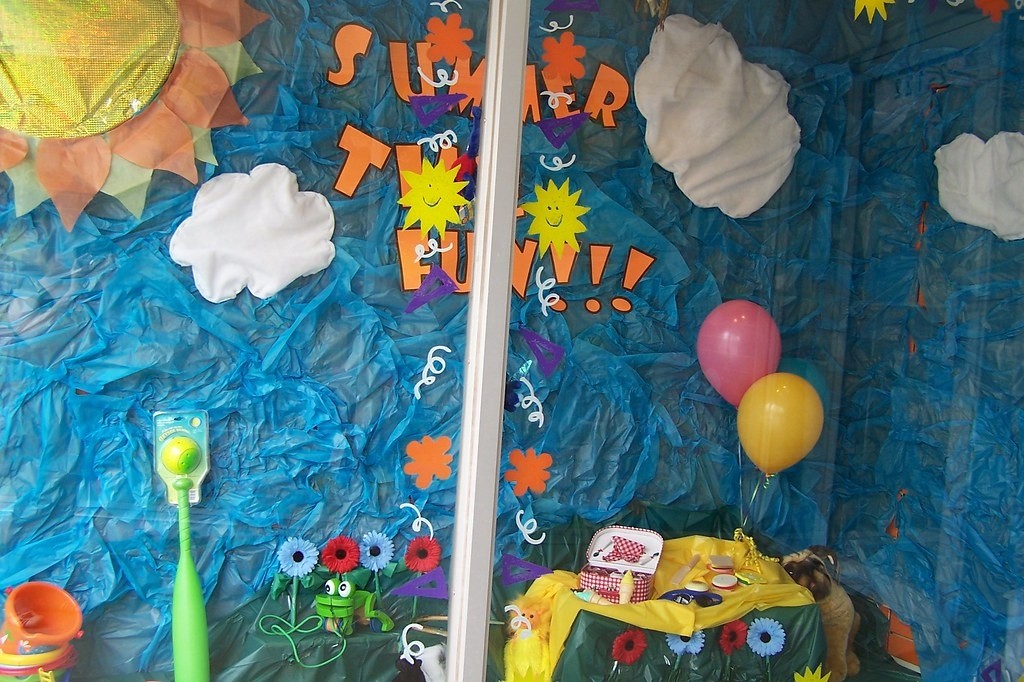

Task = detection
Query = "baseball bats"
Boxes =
[172,477,210,682]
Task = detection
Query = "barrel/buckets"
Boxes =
[0,583,82,682]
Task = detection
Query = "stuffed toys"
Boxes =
[503,598,553,682]
[776,545,862,682]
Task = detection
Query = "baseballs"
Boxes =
[160,436,203,474]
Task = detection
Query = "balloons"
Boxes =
[738,373,824,476]
[697,299,781,409]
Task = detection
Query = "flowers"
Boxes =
[394,536,442,619]
[350,529,398,611]
[665,630,706,682]
[270,536,326,633]
[746,617,787,682]
[316,536,364,586]
[719,619,748,669]
[607,628,648,682]
